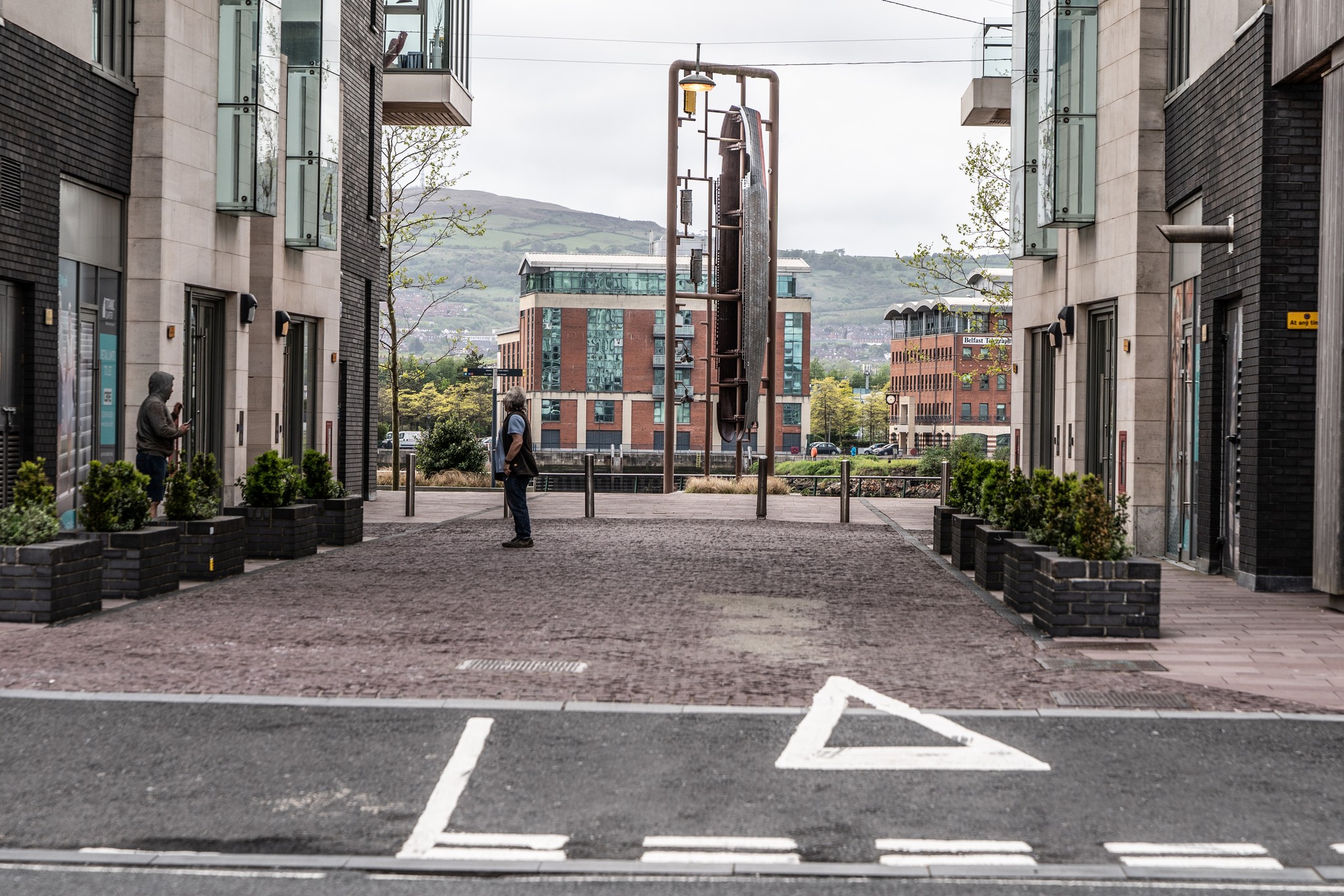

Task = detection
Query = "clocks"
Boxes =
[887,395,895,403]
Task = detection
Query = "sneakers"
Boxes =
[502,535,534,548]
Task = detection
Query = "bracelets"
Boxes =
[504,458,512,464]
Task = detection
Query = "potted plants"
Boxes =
[157,451,246,581]
[934,452,1161,640]
[224,450,317,559]
[75,459,181,600]
[0,457,103,624]
[294,448,363,545]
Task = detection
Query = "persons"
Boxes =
[495,386,538,548]
[850,444,856,456]
[136,372,190,517]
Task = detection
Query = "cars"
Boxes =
[809,442,841,456]
[469,436,499,446]
[863,443,907,455]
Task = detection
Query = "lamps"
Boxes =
[1048,322,1062,348]
[240,293,257,323]
[1057,305,1073,336]
[276,310,290,337]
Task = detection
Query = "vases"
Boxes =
[432,29,441,68]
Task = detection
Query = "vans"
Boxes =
[382,431,429,450]
